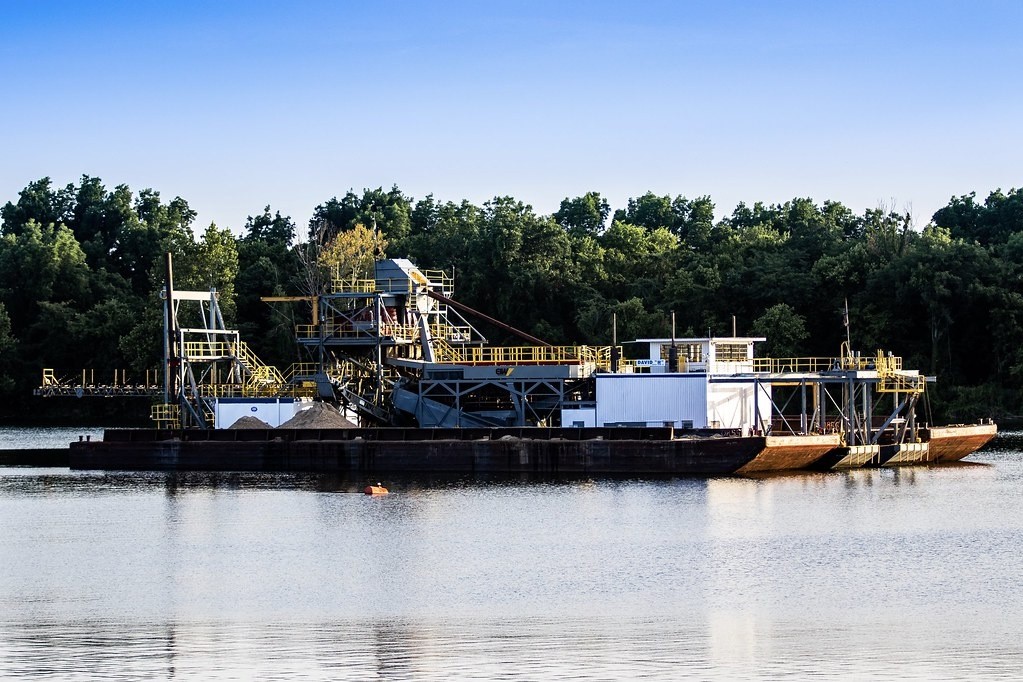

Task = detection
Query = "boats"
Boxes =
[374,258,989,464]
[70,253,841,469]
[259,273,880,465]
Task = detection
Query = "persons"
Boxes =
[333,358,342,374]
[536,414,546,427]
[392,371,402,382]
